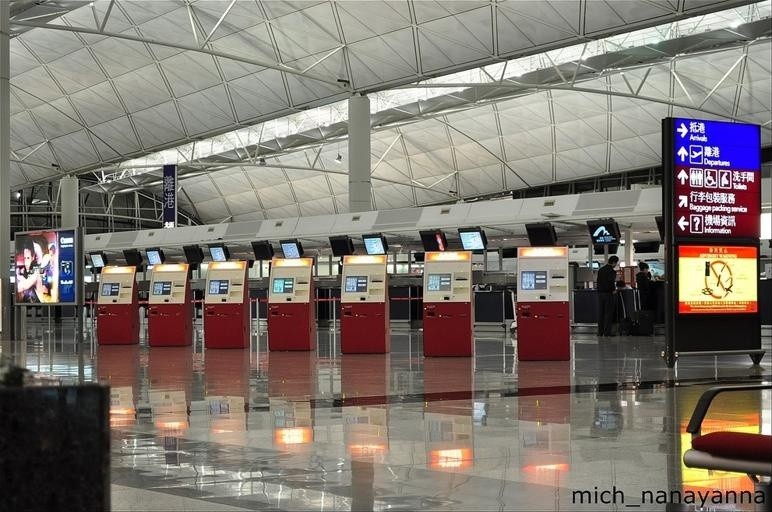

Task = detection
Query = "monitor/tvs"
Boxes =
[587,221,621,244]
[279,240,304,259]
[520,270,548,291]
[123,249,143,266]
[526,223,557,247]
[419,231,448,251]
[426,274,452,291]
[251,241,275,261]
[145,248,166,265]
[207,243,230,262]
[14,228,77,306]
[89,252,108,267]
[329,235,354,257]
[362,234,389,255]
[183,245,204,263]
[459,228,488,251]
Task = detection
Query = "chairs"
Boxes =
[683,382,772,512]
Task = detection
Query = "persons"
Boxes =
[43,245,60,302]
[616,280,633,290]
[636,262,653,314]
[31,236,52,303]
[597,256,619,336]
[16,239,38,303]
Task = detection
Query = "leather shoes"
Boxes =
[604,332,616,337]
[597,331,604,336]
